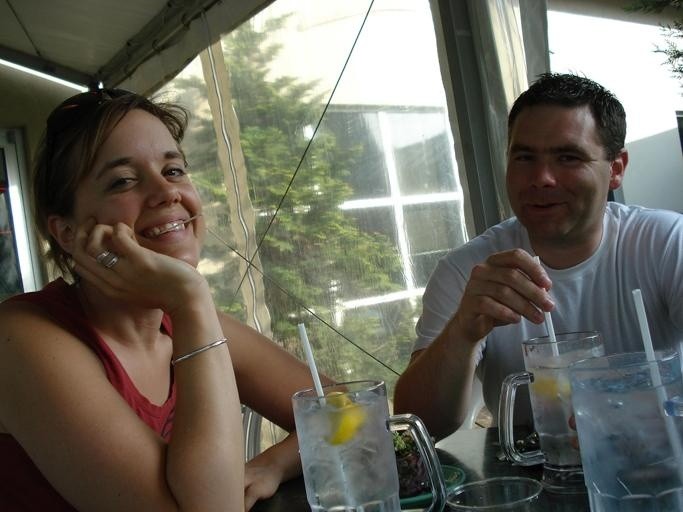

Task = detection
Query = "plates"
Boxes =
[398,462,462,507]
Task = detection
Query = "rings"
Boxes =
[96,249,118,269]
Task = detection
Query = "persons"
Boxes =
[394,76,683,444]
[1,90,347,512]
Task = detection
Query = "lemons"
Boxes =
[322,390,364,447]
[528,378,573,402]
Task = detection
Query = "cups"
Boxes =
[445,475,546,512]
[290,379,447,511]
[575,351,683,510]
[498,331,608,486]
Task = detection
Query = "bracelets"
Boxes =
[170,337,228,368]
